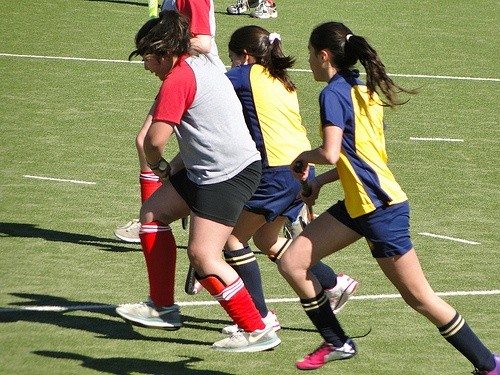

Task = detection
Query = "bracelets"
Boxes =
[146,157,163,170]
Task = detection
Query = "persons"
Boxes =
[222,25,359,336]
[117,10,282,353]
[278,22,500,375]
[226,0,278,19]
[112,0,227,242]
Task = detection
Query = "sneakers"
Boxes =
[113,219,142,242]
[115,296,182,327]
[295,337,357,370]
[250,1,278,19]
[471,354,500,375]
[222,310,281,335]
[323,273,359,315]
[213,324,281,352]
[227,0,263,15]
